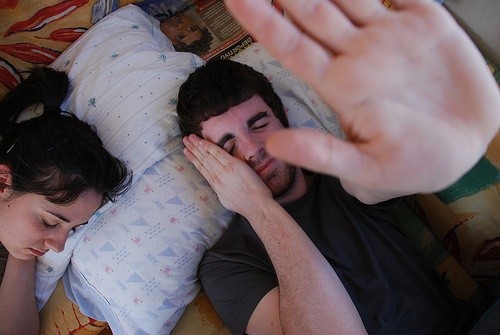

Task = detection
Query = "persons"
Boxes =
[0,66,133,335]
[136,0,211,53]
[177,0,500,335]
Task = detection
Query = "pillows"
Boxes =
[13,2,237,335]
[231,44,347,183]
